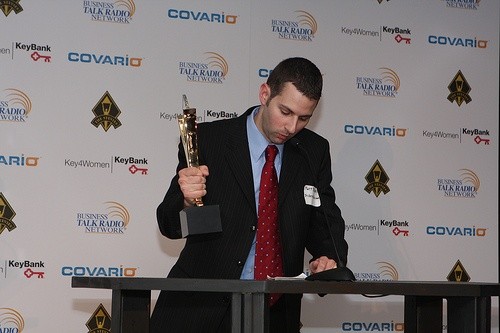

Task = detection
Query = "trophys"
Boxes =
[175,91,223,239]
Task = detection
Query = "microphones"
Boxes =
[291,138,357,282]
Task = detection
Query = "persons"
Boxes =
[148,56,348,333]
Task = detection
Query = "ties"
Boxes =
[254,145,282,308]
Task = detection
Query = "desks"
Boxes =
[71,276,500,333]
